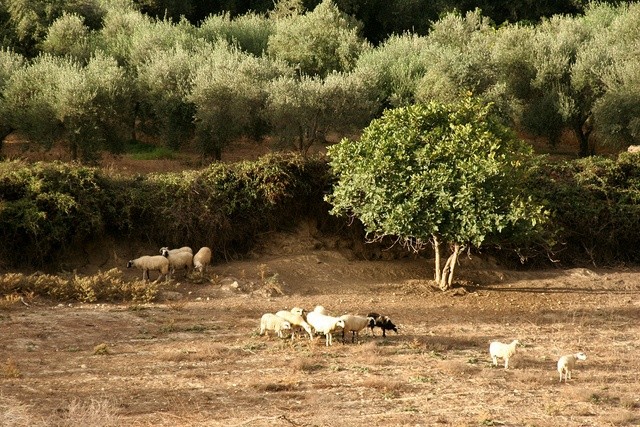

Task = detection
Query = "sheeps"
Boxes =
[490,340,523,370]
[367,313,401,339]
[160,249,194,280]
[292,307,309,322]
[341,315,377,346]
[276,311,316,341]
[193,247,213,278]
[260,314,294,340]
[315,306,329,316]
[307,312,347,347]
[558,352,588,384]
[127,256,171,284]
[160,247,193,255]
